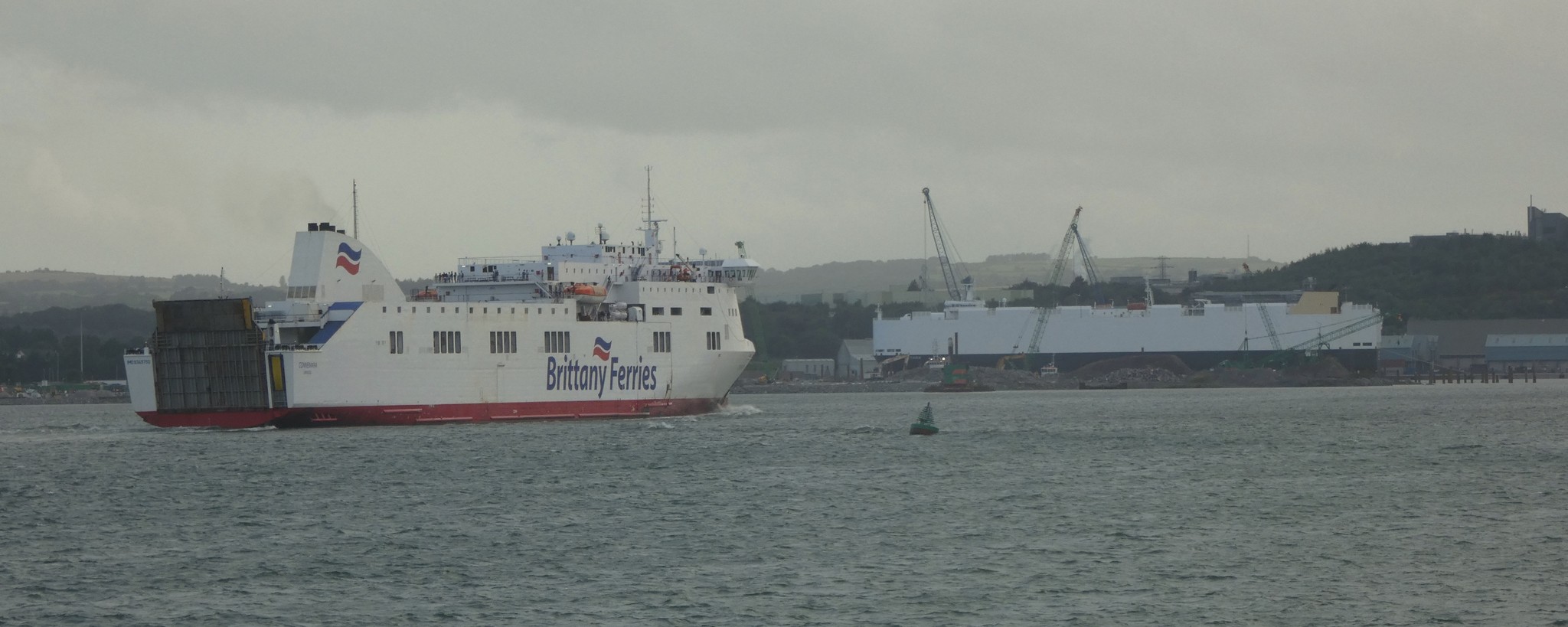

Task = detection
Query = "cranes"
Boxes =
[1241,279,1402,371]
[921,188,975,306]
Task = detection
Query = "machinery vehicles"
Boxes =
[995,205,1084,371]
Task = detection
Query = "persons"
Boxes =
[492,270,499,282]
[540,269,544,281]
[522,269,525,281]
[607,275,611,284]
[434,270,464,283]
[525,270,529,281]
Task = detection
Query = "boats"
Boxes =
[414,289,438,302]
[908,401,939,436]
[121,163,760,432]
[563,283,608,305]
[871,268,1385,379]
